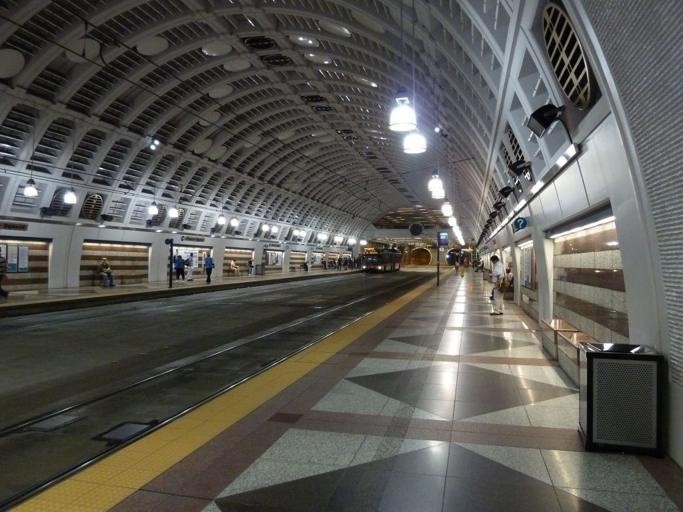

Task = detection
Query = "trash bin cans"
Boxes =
[577,342,670,457]
[256,264,265,275]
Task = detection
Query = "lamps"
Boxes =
[271,202,278,234]
[293,201,300,237]
[230,180,240,228]
[301,210,306,237]
[360,235,366,245]
[317,214,327,240]
[217,149,227,225]
[333,227,344,243]
[25,104,40,199]
[64,110,77,205]
[261,197,270,232]
[166,142,180,218]
[349,232,357,244]
[148,131,161,217]
[386,3,466,246]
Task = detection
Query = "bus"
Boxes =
[361,238,403,274]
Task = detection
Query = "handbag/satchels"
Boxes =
[496,277,511,293]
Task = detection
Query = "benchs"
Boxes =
[540,318,600,389]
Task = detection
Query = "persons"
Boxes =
[474,260,485,272]
[248,257,254,275]
[0,248,9,299]
[205,253,215,283]
[96,257,115,287]
[175,255,186,278]
[454,259,460,274]
[187,253,194,280]
[490,255,506,315]
[231,259,240,276]
[306,256,359,273]
[490,266,513,300]
